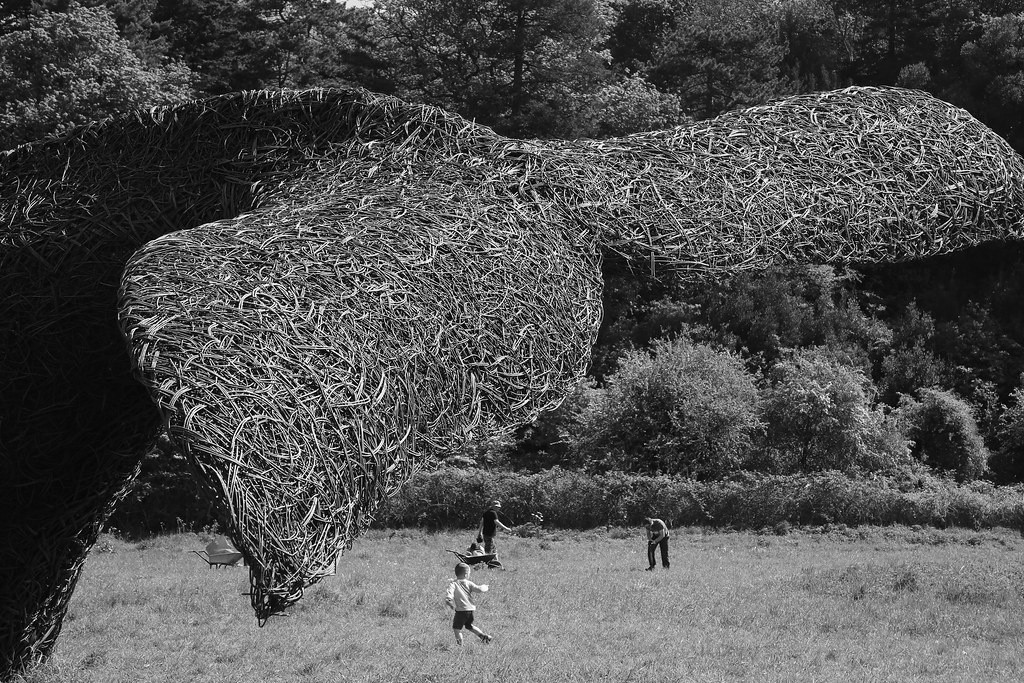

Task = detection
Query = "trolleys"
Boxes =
[446,549,502,571]
[188,550,244,569]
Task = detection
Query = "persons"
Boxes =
[445,562,493,648]
[471,541,486,556]
[478,500,512,561]
[643,517,670,572]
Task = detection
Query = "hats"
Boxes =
[489,501,501,508]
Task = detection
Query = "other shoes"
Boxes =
[481,634,492,644]
[645,566,655,571]
